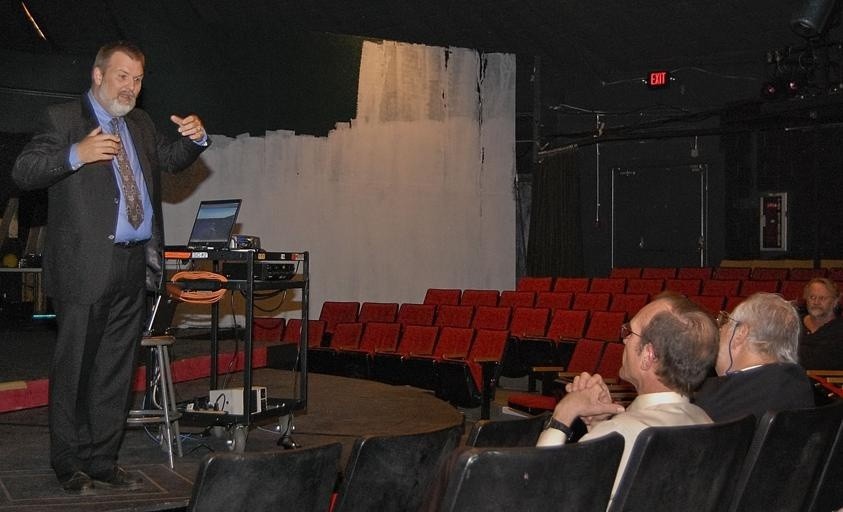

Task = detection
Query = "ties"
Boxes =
[109,116,145,231]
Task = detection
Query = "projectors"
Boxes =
[229,234,261,250]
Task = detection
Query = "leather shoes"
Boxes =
[113,238,150,250]
[85,463,145,491]
[55,467,97,498]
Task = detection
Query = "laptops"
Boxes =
[164,198,242,251]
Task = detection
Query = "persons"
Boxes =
[692,290,816,426]
[12,36,213,495]
[537,290,720,511]
[793,277,843,405]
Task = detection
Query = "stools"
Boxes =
[123,336,188,470]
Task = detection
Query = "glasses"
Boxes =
[620,323,653,344]
[715,308,739,329]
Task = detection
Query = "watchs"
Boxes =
[543,416,574,439]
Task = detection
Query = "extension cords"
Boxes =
[185,402,228,416]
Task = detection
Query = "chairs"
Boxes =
[186,267,843,512]
